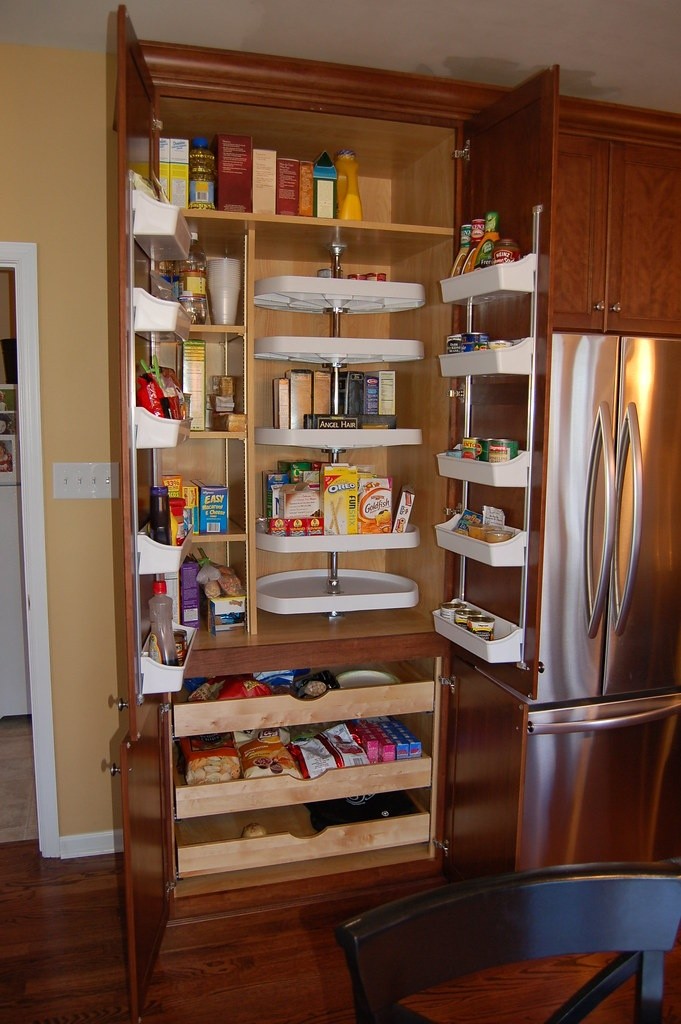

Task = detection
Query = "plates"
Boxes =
[334,668,402,689]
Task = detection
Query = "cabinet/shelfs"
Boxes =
[103,4,681,1024]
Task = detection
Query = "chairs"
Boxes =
[332,859,681,1024]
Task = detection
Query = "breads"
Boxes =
[242,823,267,838]
[211,375,246,431]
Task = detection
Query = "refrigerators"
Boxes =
[516,332,681,870]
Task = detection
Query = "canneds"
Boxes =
[317,268,386,281]
[440,602,496,641]
[491,237,521,265]
[461,436,518,464]
[459,331,513,352]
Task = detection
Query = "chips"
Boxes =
[186,755,240,785]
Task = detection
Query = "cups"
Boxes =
[207,258,240,326]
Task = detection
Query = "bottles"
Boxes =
[148,578,179,668]
[176,228,210,329]
[149,485,173,546]
[189,136,217,209]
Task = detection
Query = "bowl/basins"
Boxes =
[467,524,495,540]
[484,530,513,543]
